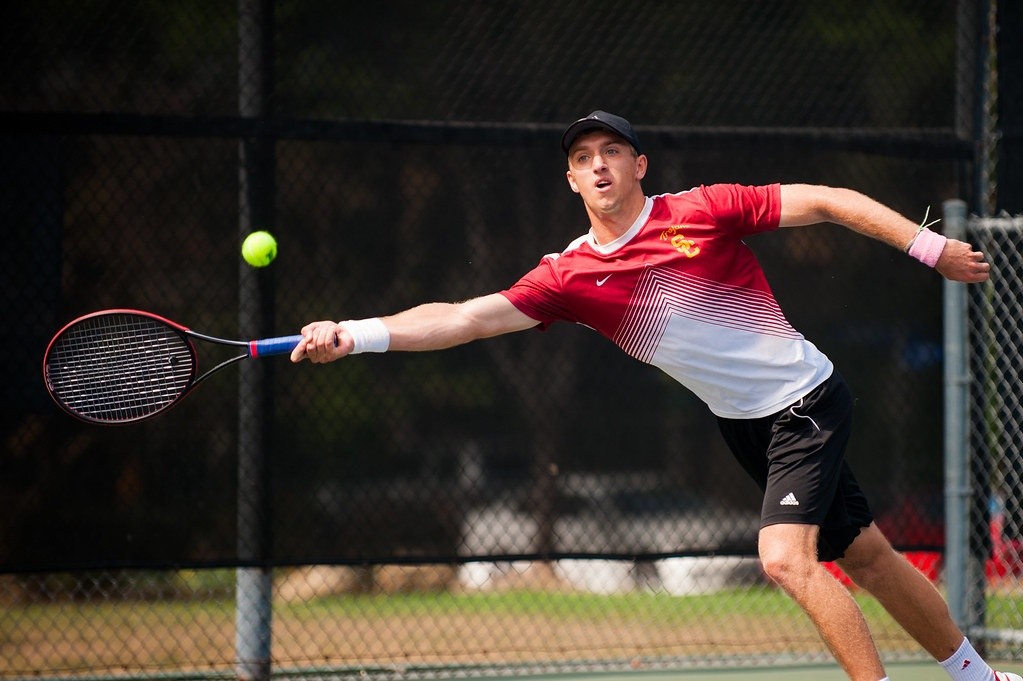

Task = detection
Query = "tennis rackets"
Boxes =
[41,307,340,426]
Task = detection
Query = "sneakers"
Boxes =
[994,670,1023,681]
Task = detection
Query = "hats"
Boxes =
[560,110,642,154]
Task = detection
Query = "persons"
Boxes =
[292,113,1023,681]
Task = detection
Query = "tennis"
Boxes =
[241,229,278,269]
